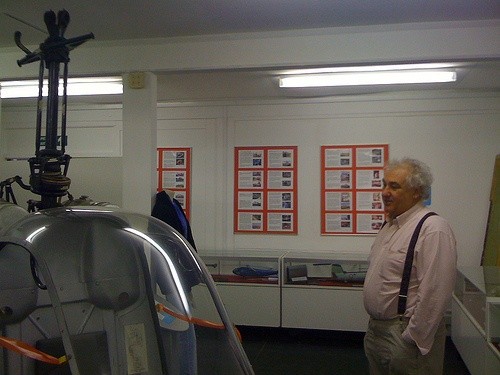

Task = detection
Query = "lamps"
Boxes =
[0,77,123,99]
[277,69,458,89]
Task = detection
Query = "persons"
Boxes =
[361,155,459,375]
[148,188,210,375]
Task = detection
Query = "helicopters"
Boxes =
[233,265,279,278]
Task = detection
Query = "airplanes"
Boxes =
[330,264,367,283]
